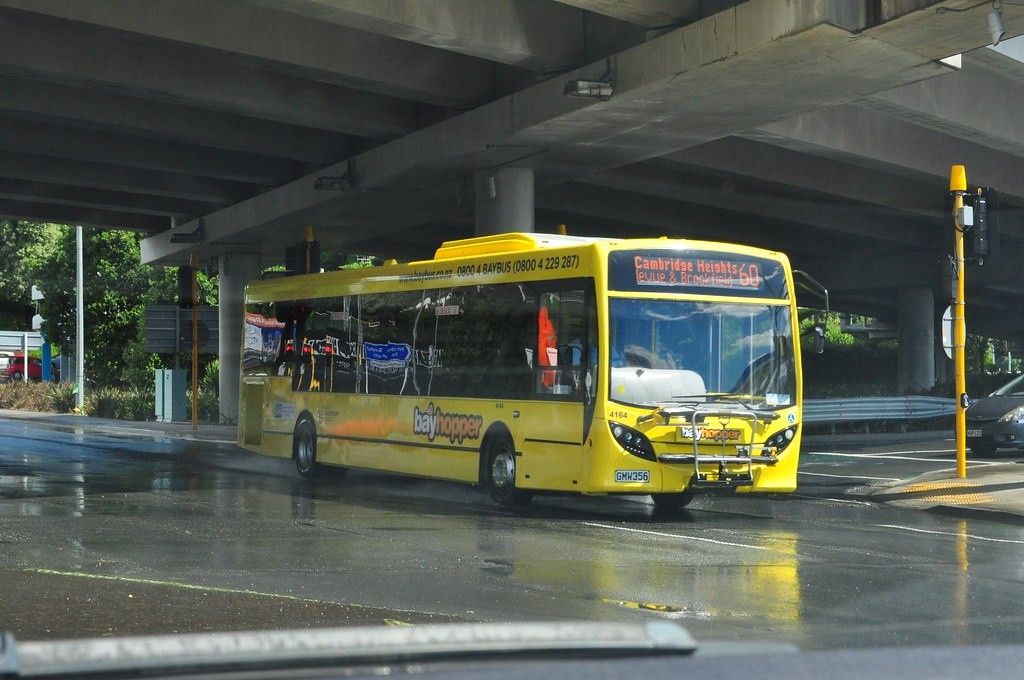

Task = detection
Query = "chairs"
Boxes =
[558,344,574,386]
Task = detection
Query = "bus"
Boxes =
[238,231,830,511]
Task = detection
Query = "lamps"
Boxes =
[562,55,616,102]
[314,158,356,193]
[170,219,204,246]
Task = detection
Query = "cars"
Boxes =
[0,351,14,371]
[954,374,1024,458]
[6,355,58,381]
[51,353,60,369]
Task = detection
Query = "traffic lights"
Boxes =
[326,335,334,367]
[285,243,304,276]
[282,330,295,364]
[300,334,312,365]
